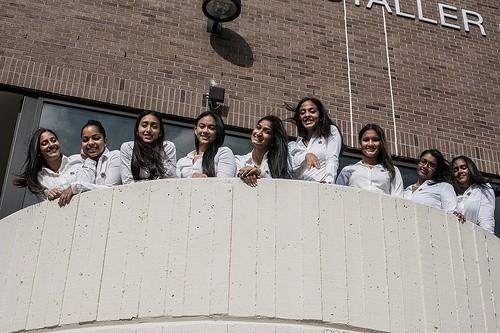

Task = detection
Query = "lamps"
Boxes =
[202,0,241,33]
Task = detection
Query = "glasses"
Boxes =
[419,158,436,169]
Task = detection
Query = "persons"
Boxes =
[12,127,85,201]
[335,123,404,199]
[120,110,177,184]
[285,97,348,184]
[403,148,457,217]
[234,114,289,187]
[58,119,123,208]
[449,154,496,234]
[175,111,237,177]
